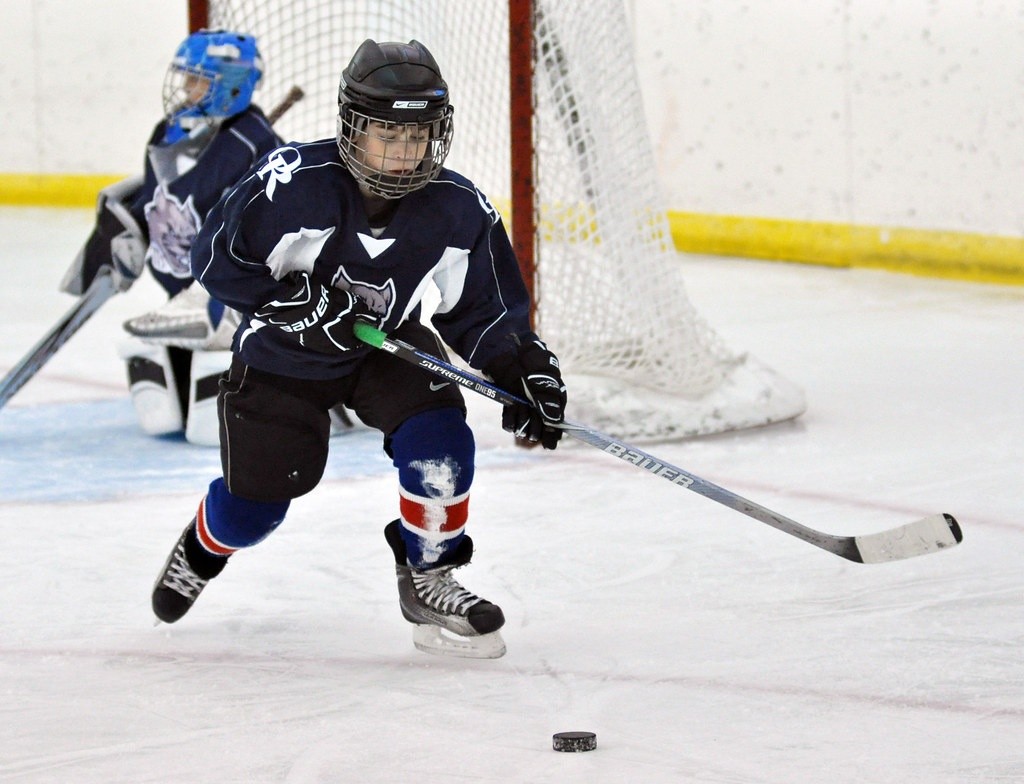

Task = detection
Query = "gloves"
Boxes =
[485,341,568,450]
[253,271,381,354]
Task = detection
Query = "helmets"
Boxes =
[337,38,454,201]
[162,30,263,141]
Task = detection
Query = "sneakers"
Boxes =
[152,516,228,625]
[384,518,507,658]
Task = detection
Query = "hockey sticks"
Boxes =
[0,84,308,412]
[354,323,965,565]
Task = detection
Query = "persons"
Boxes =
[151,41,568,637]
[110,27,378,447]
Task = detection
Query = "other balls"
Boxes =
[552,730,597,754]
